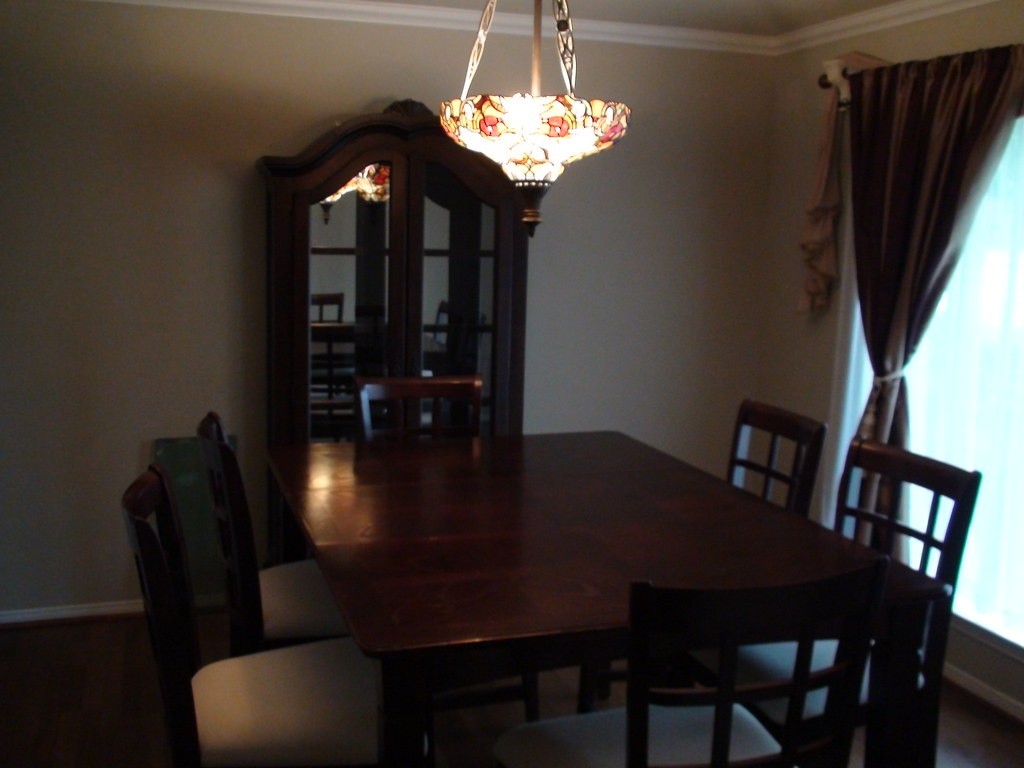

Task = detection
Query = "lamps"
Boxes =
[318,163,389,204]
[440,0,632,239]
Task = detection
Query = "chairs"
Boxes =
[495,554,891,768]
[431,300,488,352]
[121,464,429,768]
[312,292,359,439]
[198,410,350,654]
[352,372,540,729]
[579,396,827,712]
[685,431,982,767]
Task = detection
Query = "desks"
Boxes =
[312,320,449,369]
[259,428,952,768]
